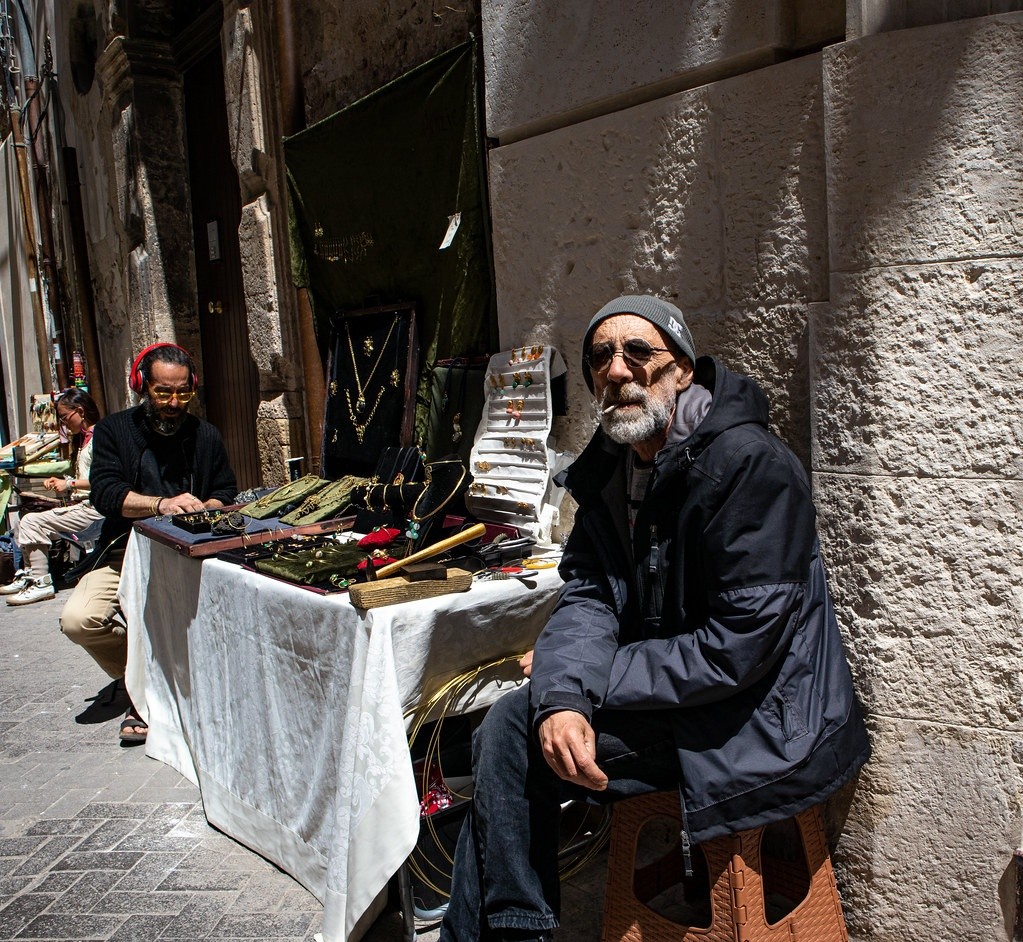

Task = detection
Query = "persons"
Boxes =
[435,294,871,942]
[58,345,238,740]
[0,388,106,605]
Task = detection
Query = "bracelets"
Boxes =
[226,508,253,530]
[261,525,320,561]
[148,497,164,516]
[66,479,76,491]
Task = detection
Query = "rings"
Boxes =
[192,497,196,500]
[329,574,357,589]
[183,510,224,523]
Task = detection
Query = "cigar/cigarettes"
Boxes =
[601,403,621,415]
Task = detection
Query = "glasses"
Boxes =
[60,409,76,423]
[147,384,195,403]
[586,338,670,372]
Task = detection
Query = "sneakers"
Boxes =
[0,569,34,594]
[6,573,55,605]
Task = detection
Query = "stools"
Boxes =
[604,783,850,942]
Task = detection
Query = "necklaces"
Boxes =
[346,317,398,414]
[345,387,385,445]
[156,427,179,437]
[253,446,466,557]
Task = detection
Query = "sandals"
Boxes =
[118,706,148,740]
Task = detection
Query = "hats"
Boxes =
[581,295,697,396]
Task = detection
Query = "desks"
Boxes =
[0,459,78,534]
[115,501,569,942]
